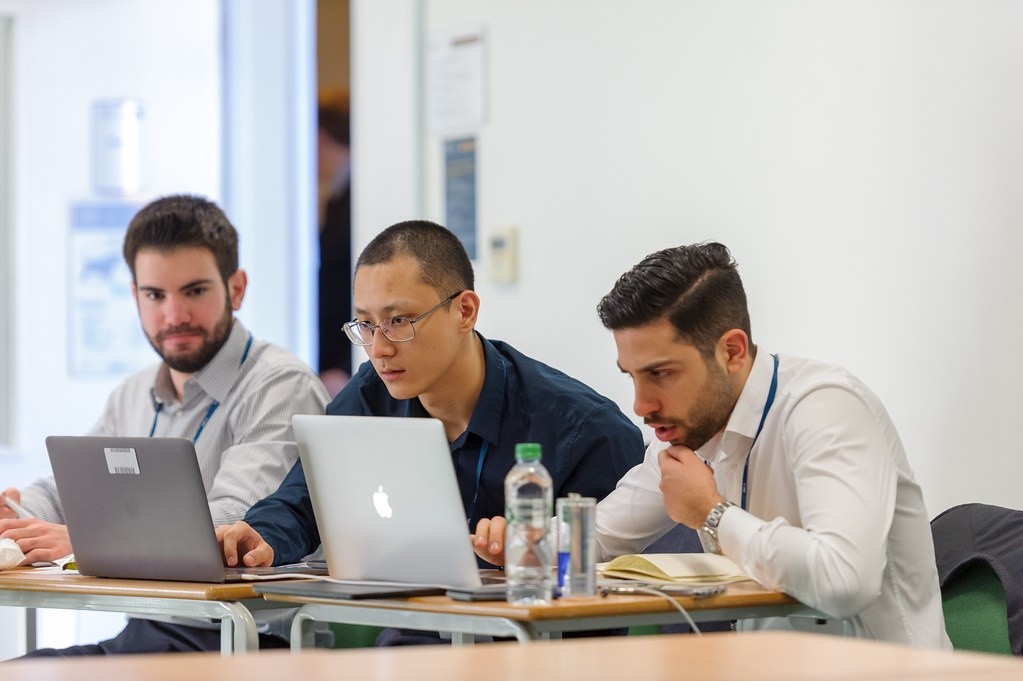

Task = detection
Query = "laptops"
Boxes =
[291,412,562,594]
[45,436,329,582]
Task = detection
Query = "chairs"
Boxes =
[930,503,1023,656]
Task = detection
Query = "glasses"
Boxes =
[341,290,459,346]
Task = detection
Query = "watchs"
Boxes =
[700,501,738,554]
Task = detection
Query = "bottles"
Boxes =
[503,442,555,608]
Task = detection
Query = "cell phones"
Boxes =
[446,588,539,603]
[598,581,726,598]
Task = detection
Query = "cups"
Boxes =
[556,496,599,605]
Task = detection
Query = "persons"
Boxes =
[318,104,351,399]
[214,221,646,647]
[0,194,333,663]
[468,241,954,651]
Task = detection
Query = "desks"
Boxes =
[0,567,790,655]
[0,628,1022,681]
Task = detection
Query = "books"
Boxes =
[595,553,751,585]
